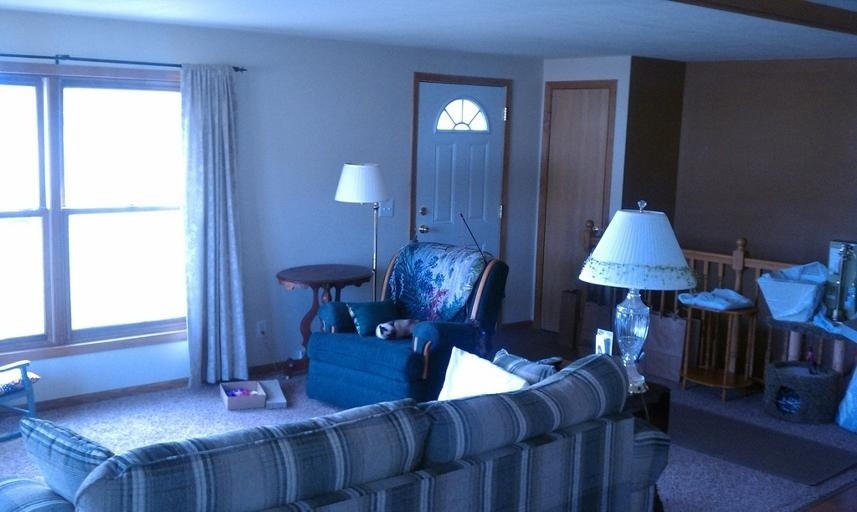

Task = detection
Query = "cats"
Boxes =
[375,318,422,341]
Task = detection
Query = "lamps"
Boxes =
[579,199,698,395]
[334,161,391,303]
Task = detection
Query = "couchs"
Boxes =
[0,353,671,511]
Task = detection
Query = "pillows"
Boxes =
[17,415,116,503]
[346,302,399,337]
[437,345,558,403]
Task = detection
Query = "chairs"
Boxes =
[307,241,510,410]
[0,358,42,444]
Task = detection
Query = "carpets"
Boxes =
[0,331,857,512]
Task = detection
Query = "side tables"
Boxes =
[681,306,759,403]
[621,380,670,434]
[276,263,374,374]
